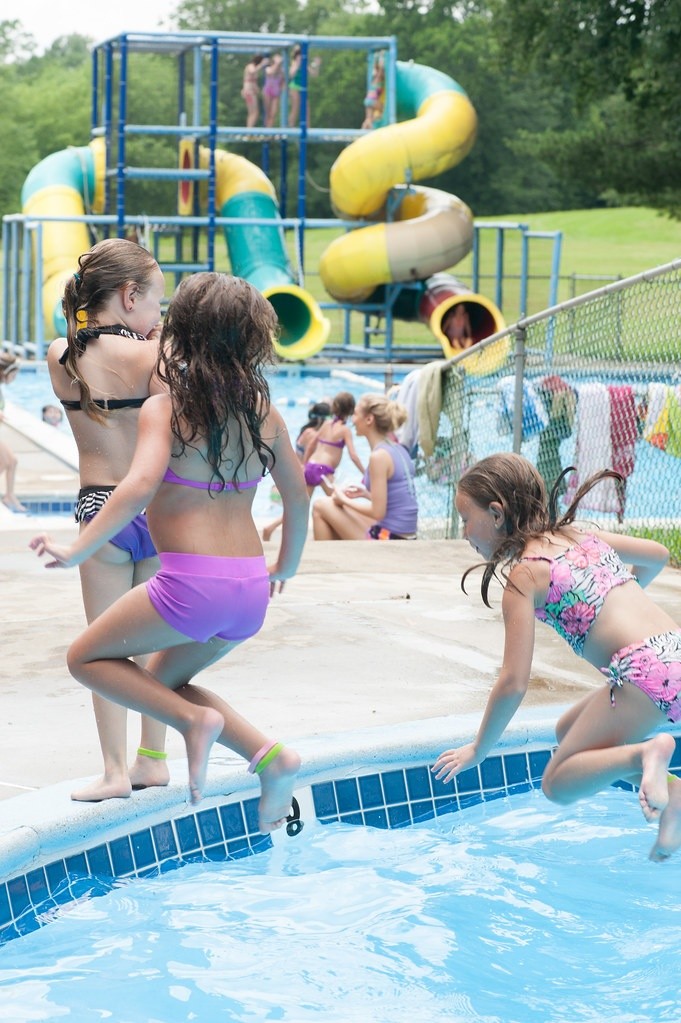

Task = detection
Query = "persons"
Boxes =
[261,54,284,127]
[30,271,309,835]
[0,352,26,512]
[360,50,383,129]
[295,401,331,465]
[312,393,419,541]
[441,304,473,352]
[288,45,321,127]
[241,56,269,127]
[431,451,680,862]
[42,405,62,427]
[262,393,366,541]
[47,238,175,800]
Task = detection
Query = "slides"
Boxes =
[321,59,517,384]
[175,137,331,363]
[20,134,110,351]
